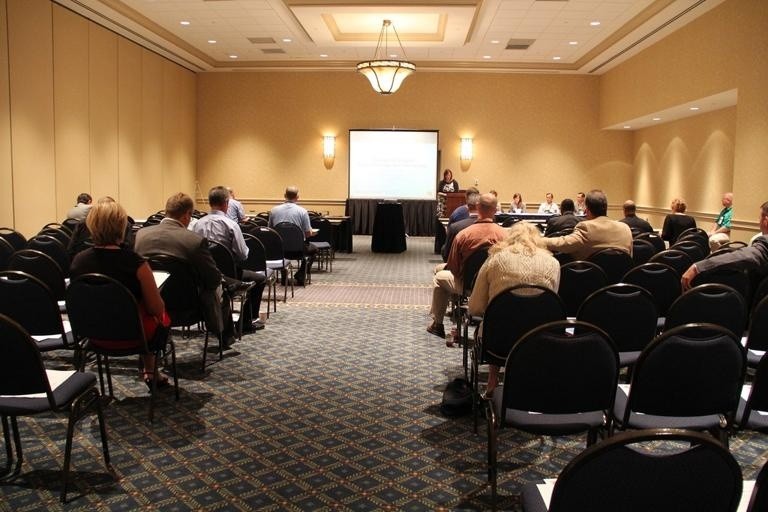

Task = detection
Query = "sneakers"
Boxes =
[427,321,445,338]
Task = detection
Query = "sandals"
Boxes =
[144,372,169,390]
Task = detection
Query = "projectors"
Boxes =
[382,198,397,204]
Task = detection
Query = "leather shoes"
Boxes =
[239,323,265,331]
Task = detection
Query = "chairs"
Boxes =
[65,273,181,426]
[41,222,74,234]
[558,260,610,316]
[669,241,707,263]
[486,319,620,499]
[309,217,332,273]
[749,276,768,350]
[0,314,116,505]
[38,228,71,246]
[191,209,208,219]
[452,248,488,348]
[237,222,257,233]
[735,351,768,432]
[0,227,27,248]
[547,228,574,239]
[141,249,223,373]
[616,261,681,282]
[471,283,568,435]
[143,210,166,228]
[273,222,311,289]
[242,232,277,319]
[521,428,744,512]
[206,240,257,342]
[665,283,749,341]
[27,234,66,249]
[250,226,294,303]
[611,324,747,450]
[574,282,660,383]
[633,238,656,261]
[63,218,80,225]
[9,249,64,285]
[249,218,268,227]
[679,234,710,255]
[648,247,692,274]
[587,247,635,282]
[701,242,748,259]
[0,270,105,400]
[677,227,709,242]
[0,236,13,271]
[257,212,269,218]
[634,231,666,254]
[743,460,768,512]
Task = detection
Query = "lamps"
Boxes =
[322,136,336,159]
[460,138,473,161]
[357,19,417,97]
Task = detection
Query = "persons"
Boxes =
[439,169,459,193]
[574,192,588,212]
[448,186,485,216]
[66,192,96,223]
[267,186,317,287]
[618,200,654,235]
[467,221,562,400]
[426,193,509,337]
[542,189,633,260]
[221,188,251,225]
[546,200,589,239]
[660,198,697,248]
[679,202,768,292]
[537,193,561,214]
[134,192,235,346]
[506,193,526,214]
[707,192,733,237]
[441,195,485,260]
[192,186,265,332]
[488,191,502,214]
[67,200,173,396]
[66,195,134,255]
